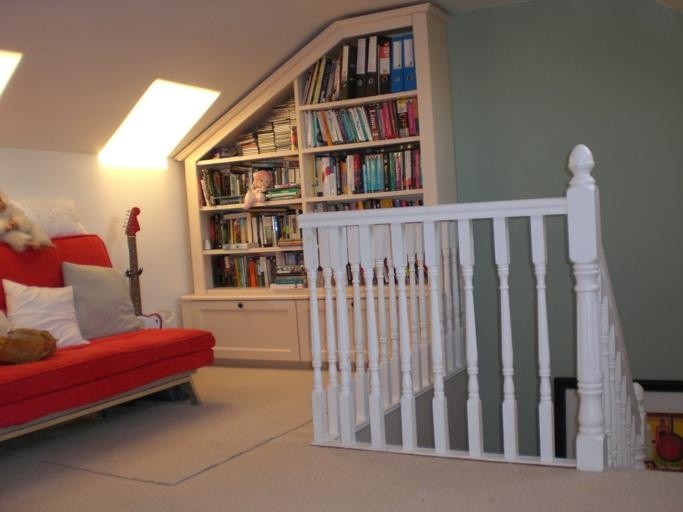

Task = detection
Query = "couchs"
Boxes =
[0,228,219,454]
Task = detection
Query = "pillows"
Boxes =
[0,260,149,368]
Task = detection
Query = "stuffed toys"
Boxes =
[0,193,59,254]
[241,170,273,211]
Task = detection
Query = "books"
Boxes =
[209,205,303,249]
[316,232,428,287]
[200,95,298,160]
[312,141,423,212]
[211,250,307,288]
[302,55,340,105]
[303,94,420,148]
[199,157,302,207]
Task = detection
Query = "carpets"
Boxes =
[7,401,311,487]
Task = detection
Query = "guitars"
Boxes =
[123,207,162,329]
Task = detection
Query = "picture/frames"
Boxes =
[548,376,683,475]
